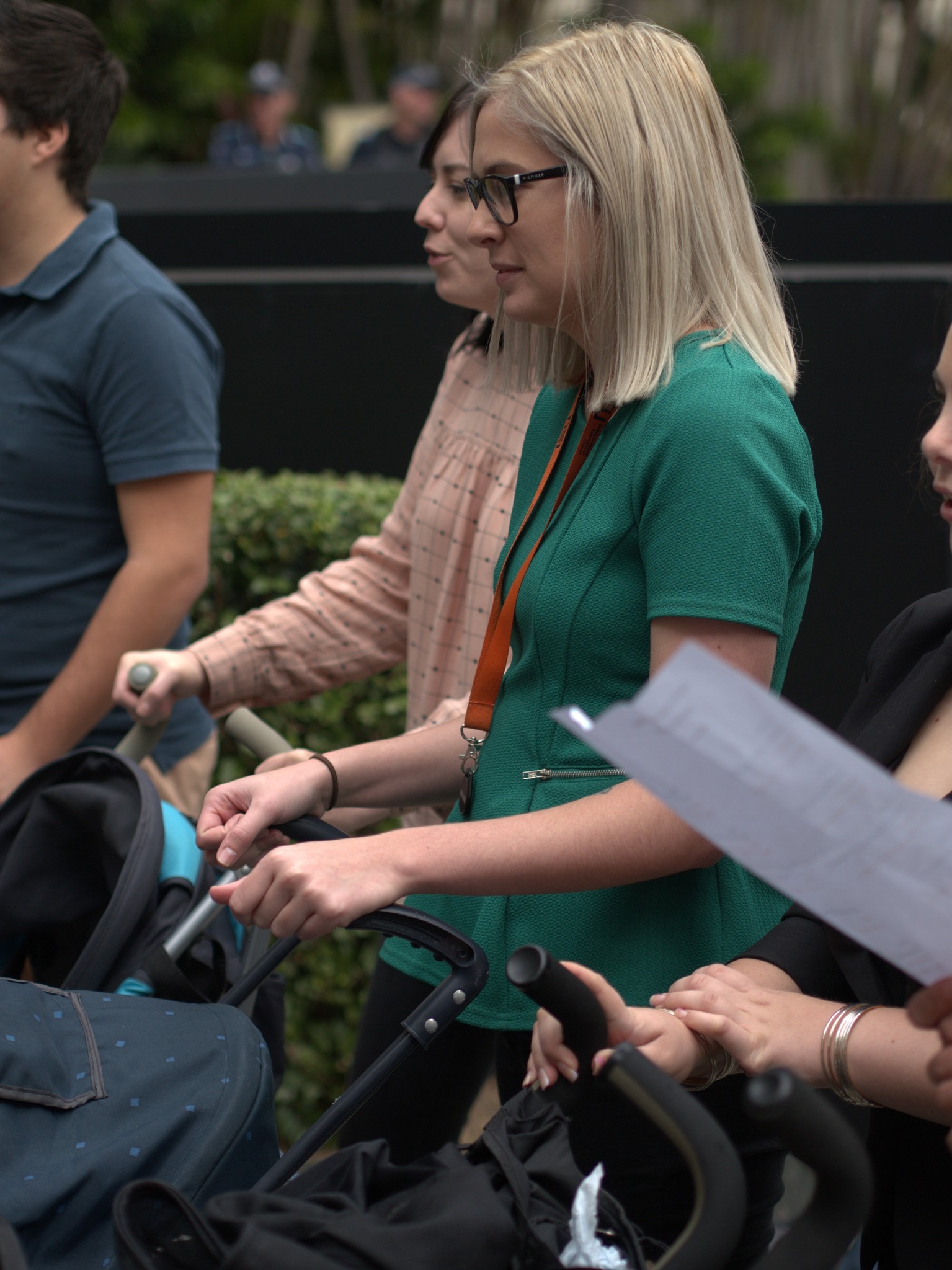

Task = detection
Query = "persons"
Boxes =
[202,21,822,1157]
[209,60,449,176]
[0,0,217,817]
[111,47,552,1144]
[520,326,952,1270]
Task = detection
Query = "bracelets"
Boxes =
[818,1002,888,1109]
[309,754,338,811]
[659,1007,737,1093]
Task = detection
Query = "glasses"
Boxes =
[464,164,575,226]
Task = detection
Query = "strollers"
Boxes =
[0,656,496,1270]
[112,942,875,1269]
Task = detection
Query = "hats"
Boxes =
[244,63,298,94]
[396,64,449,93]
